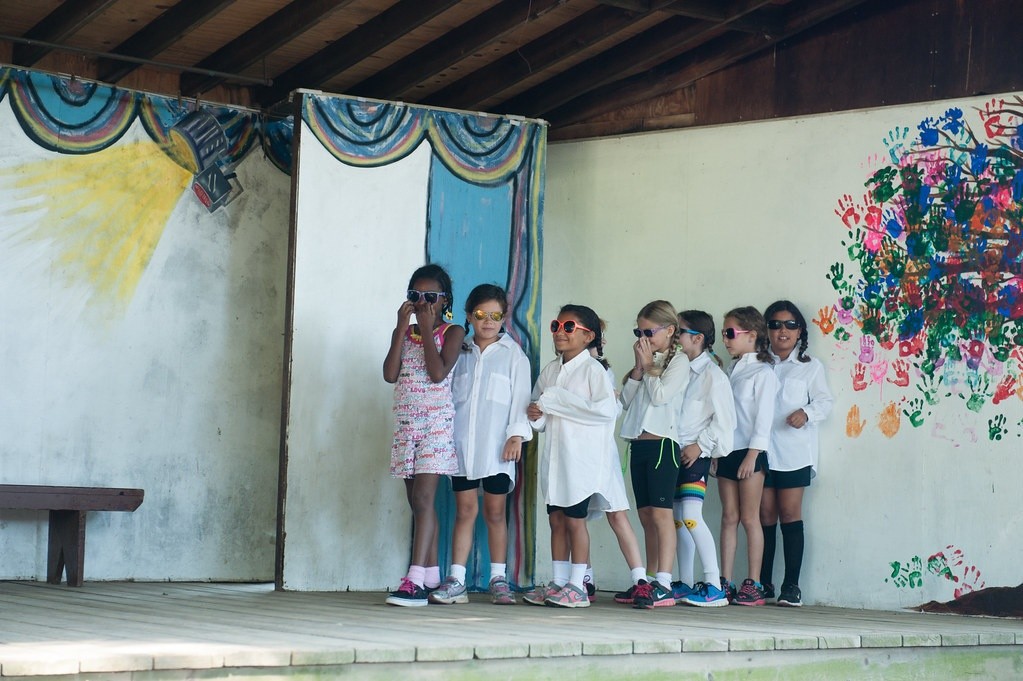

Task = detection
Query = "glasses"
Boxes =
[633,326,665,338]
[680,328,700,335]
[767,320,800,329]
[472,310,504,322]
[721,327,748,339]
[406,290,446,306]
[550,320,591,335]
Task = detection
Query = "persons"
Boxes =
[427,280,533,605]
[670,310,738,607]
[708,300,834,607]
[620,299,690,609]
[581,318,649,603]
[523,304,617,608]
[383,261,466,605]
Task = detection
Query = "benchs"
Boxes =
[0,483,144,587]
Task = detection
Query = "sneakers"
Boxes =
[731,579,766,606]
[583,575,596,602]
[489,576,516,604]
[385,577,428,606]
[544,582,591,608]
[670,580,699,603]
[522,580,563,605]
[632,579,654,609]
[428,575,469,604]
[763,583,776,604]
[649,581,675,607]
[679,581,729,607]
[720,576,737,604]
[423,584,441,597]
[614,585,638,603]
[777,584,803,606]
